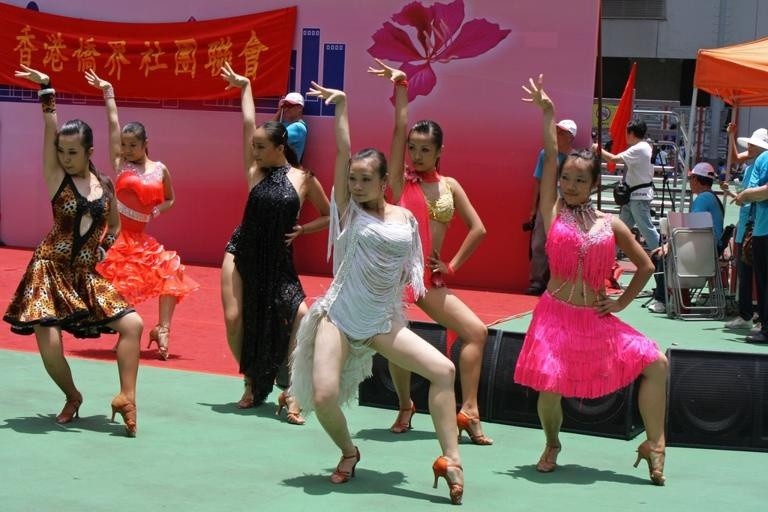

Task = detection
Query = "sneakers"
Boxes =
[527,288,543,294]
[725,318,753,329]
[648,301,666,313]
[746,332,767,343]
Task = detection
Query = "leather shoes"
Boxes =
[750,322,761,331]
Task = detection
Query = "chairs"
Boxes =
[643,211,735,321]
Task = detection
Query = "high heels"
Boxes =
[391,401,416,433]
[433,456,464,505]
[111,393,137,437]
[275,392,305,425]
[457,412,494,445]
[536,445,562,472]
[56,392,82,424]
[330,446,360,484]
[633,441,665,486]
[239,383,256,409]
[148,324,170,360]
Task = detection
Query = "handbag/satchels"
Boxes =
[740,229,754,265]
[614,181,630,205]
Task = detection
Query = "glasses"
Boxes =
[281,104,299,109]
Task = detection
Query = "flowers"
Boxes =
[365,2,511,107]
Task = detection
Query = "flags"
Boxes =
[608,63,637,175]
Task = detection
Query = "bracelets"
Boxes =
[152,206,161,219]
[103,88,115,99]
[392,78,409,90]
[36,88,56,96]
[300,224,306,237]
[444,261,455,275]
[98,245,107,258]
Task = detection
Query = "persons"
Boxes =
[85,67,202,362]
[2,65,144,439]
[724,123,766,344]
[366,55,496,446]
[285,77,467,505]
[592,119,660,257]
[649,162,724,314]
[523,117,578,297]
[519,71,670,486]
[218,59,333,426]
[270,91,308,169]
[656,144,669,176]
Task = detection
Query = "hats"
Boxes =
[555,120,577,138]
[737,128,768,150]
[280,92,305,107]
[688,162,714,179]
